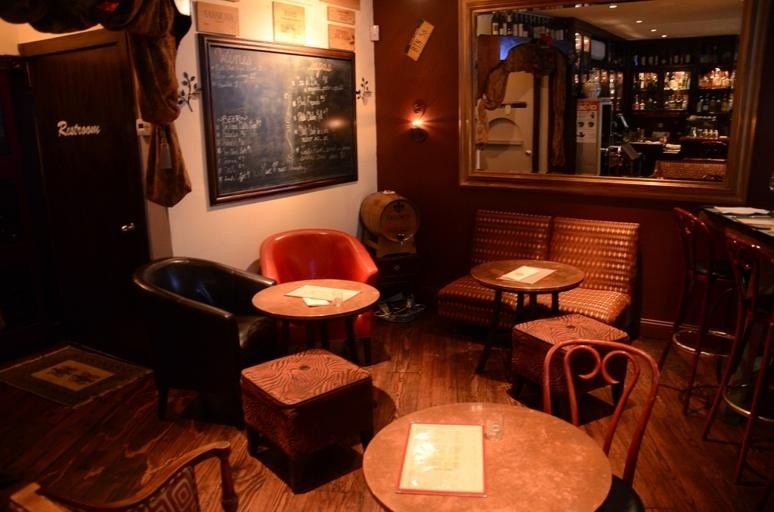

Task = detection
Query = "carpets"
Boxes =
[0,339,154,409]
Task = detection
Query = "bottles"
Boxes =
[574,32,623,99]
[492,11,564,41]
[690,67,736,140]
[631,54,692,111]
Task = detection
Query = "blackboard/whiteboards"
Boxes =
[197,32,359,207]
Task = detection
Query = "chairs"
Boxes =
[655,205,723,417]
[542,338,661,511]
[698,228,774,484]
[2,439,239,512]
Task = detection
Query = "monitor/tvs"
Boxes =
[615,113,630,131]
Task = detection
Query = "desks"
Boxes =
[361,400,614,511]
[473,257,586,373]
[251,278,382,368]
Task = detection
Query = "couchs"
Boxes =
[258,228,379,354]
[128,254,277,431]
[434,206,551,339]
[517,216,641,350]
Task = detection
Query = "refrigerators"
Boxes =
[576,101,614,176]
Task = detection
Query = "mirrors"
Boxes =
[456,1,766,208]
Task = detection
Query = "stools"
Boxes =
[240,345,375,495]
[508,312,632,411]
